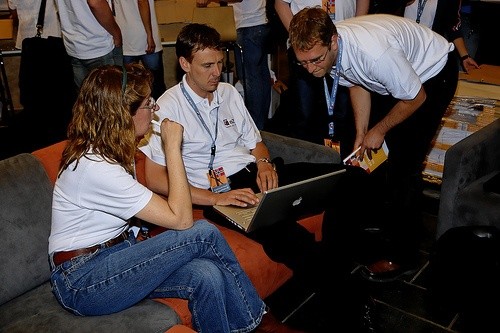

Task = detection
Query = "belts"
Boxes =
[47,231,129,271]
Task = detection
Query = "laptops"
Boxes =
[213,169,347,234]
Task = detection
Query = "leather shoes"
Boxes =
[360,257,418,283]
[255,305,305,333]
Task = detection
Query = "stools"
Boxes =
[189,5,247,113]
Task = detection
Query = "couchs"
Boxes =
[435,119,500,242]
[0,130,342,333]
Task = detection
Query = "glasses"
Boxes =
[139,96,157,111]
[296,43,330,66]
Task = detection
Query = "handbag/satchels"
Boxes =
[18,36,60,110]
[423,225,499,311]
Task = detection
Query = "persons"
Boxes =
[288,7,460,284]
[107,0,166,101]
[195,0,275,130]
[54,0,125,96]
[137,22,375,304]
[274,0,369,147]
[369,0,480,75]
[47,62,266,333]
[7,0,75,142]
